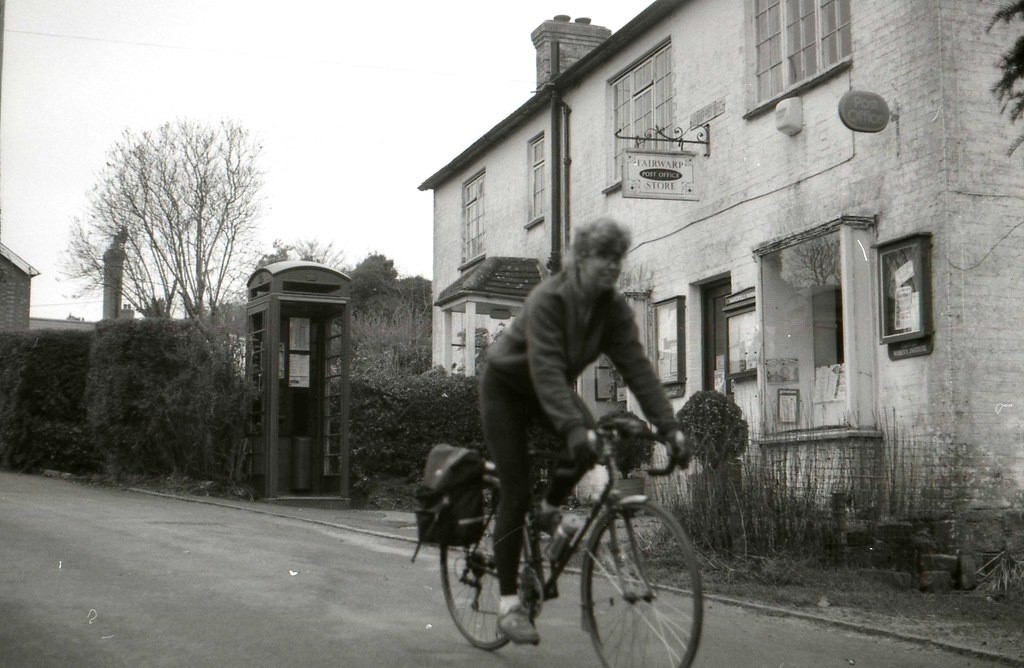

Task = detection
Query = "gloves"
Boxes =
[660,420,690,469]
[567,425,598,471]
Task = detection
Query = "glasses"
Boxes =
[586,249,624,262]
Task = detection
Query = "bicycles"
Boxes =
[439,425,703,667]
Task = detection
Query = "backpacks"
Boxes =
[414,444,485,546]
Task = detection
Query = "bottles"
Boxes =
[543,514,580,564]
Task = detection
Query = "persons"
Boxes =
[479,218,692,643]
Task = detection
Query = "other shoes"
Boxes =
[533,503,564,536]
[498,605,540,644]
